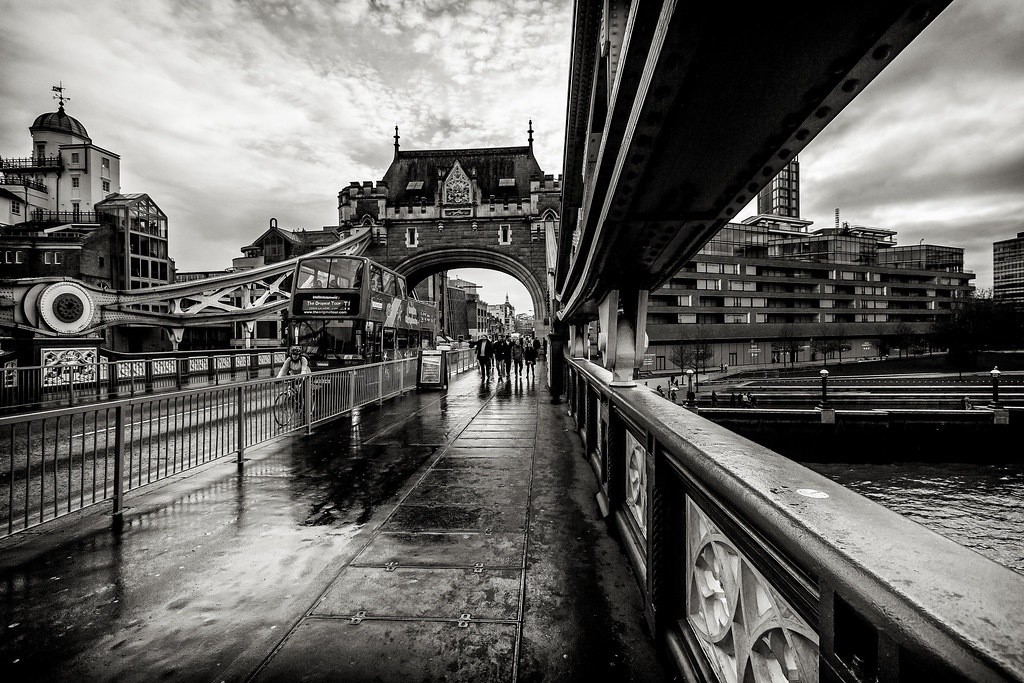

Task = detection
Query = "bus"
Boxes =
[276,254,438,391]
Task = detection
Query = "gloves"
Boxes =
[274,381,282,385]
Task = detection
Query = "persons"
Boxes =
[644,357,776,409]
[961,396,972,410]
[474,334,548,383]
[276,346,314,416]
[311,275,361,288]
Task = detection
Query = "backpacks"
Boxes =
[300,353,310,366]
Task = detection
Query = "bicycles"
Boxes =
[272,380,316,427]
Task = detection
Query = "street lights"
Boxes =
[987,365,1004,409]
[682,370,699,415]
[814,369,835,412]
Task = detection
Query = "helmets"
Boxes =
[290,347,301,354]
